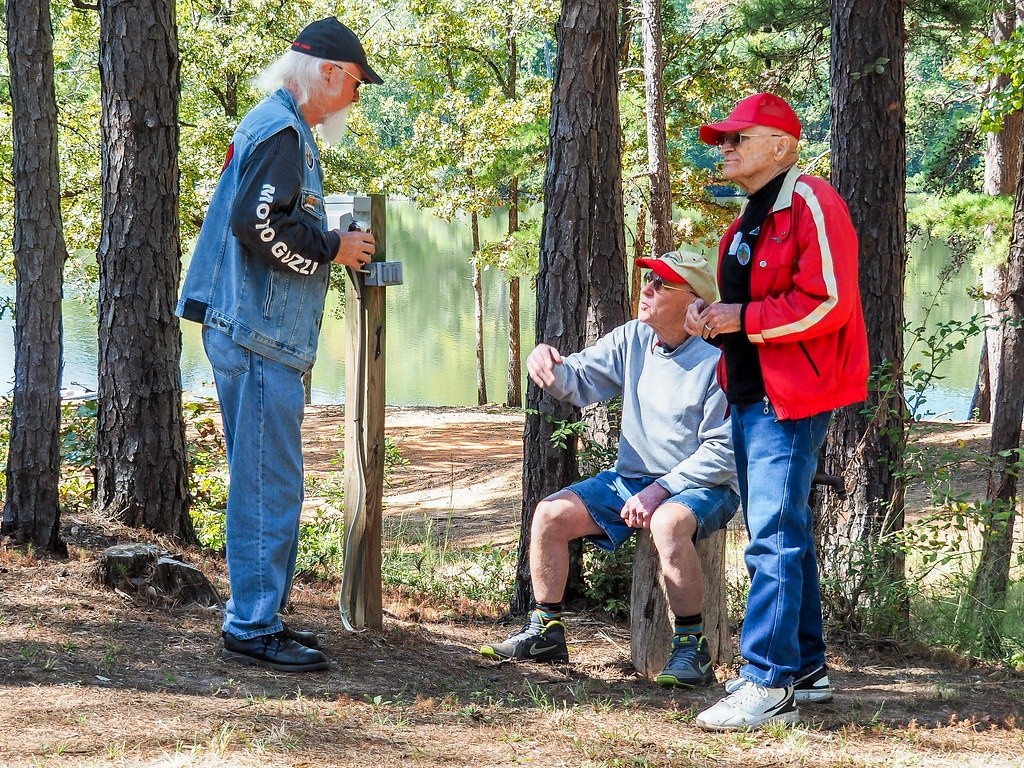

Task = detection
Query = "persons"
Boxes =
[692,92,869,732]
[175,16,375,674]
[479,250,742,691]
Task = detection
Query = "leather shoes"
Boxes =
[222,632,329,672]
[221,620,321,653]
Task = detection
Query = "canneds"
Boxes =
[348,221,372,266]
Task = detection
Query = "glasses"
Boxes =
[643,272,698,298]
[332,63,364,88]
[716,132,783,151]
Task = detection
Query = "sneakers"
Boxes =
[654,634,718,689]
[478,611,569,664]
[725,664,833,702]
[696,681,802,731]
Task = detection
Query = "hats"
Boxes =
[635,250,717,305]
[699,93,802,146]
[291,15,384,86]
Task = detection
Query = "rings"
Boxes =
[704,324,712,332]
